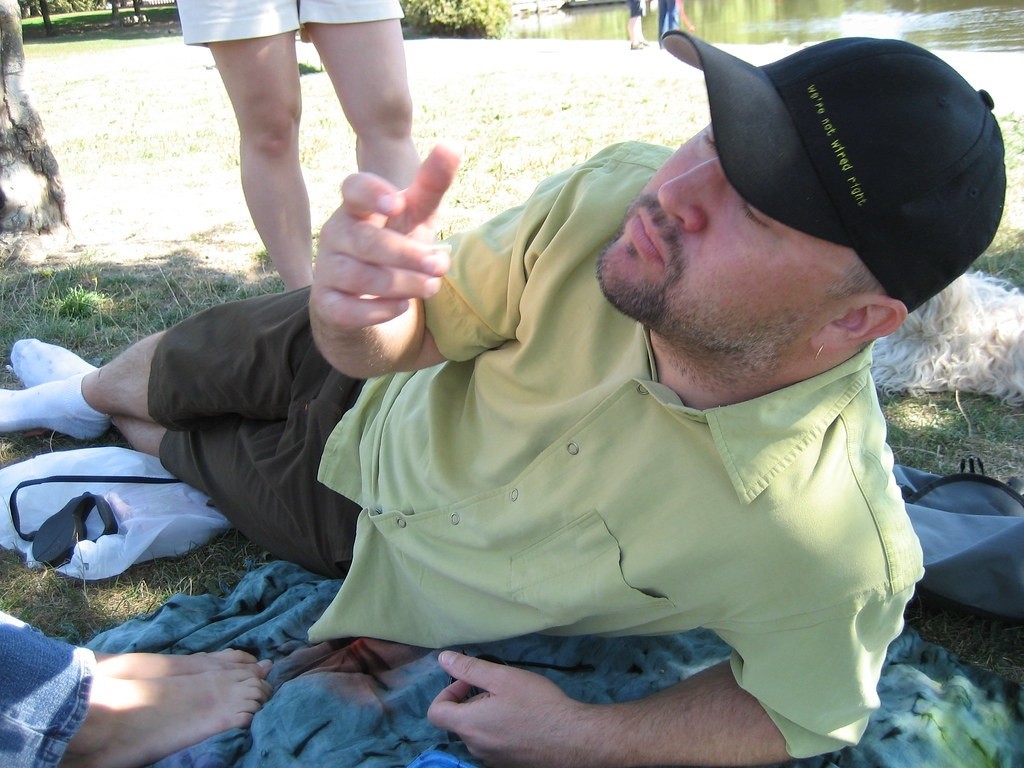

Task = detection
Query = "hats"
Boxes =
[662,29,1007,314]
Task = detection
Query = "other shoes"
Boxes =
[631,42,647,50]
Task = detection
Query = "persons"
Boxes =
[177,0,422,293]
[0,29,1007,768]
[0,609,273,768]
[658,0,680,49]
[627,0,649,49]
